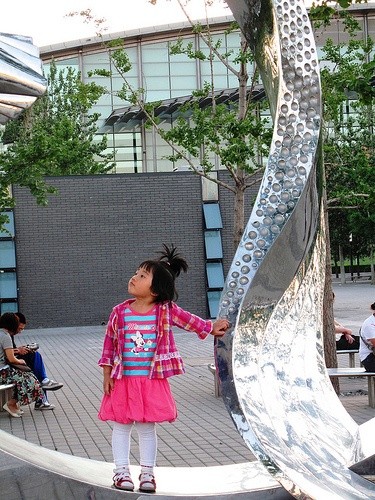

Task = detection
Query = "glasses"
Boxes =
[19,329,24,332]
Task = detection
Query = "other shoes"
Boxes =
[112,467,135,490]
[34,403,55,410]
[139,472,156,490]
[3,402,24,418]
[42,379,63,390]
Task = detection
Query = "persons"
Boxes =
[97,243,229,491]
[0,311,64,417]
[331,292,360,350]
[358,302,375,374]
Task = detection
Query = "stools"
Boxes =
[0,383,15,411]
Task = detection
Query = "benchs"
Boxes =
[327,368,375,409]
[336,350,359,380]
